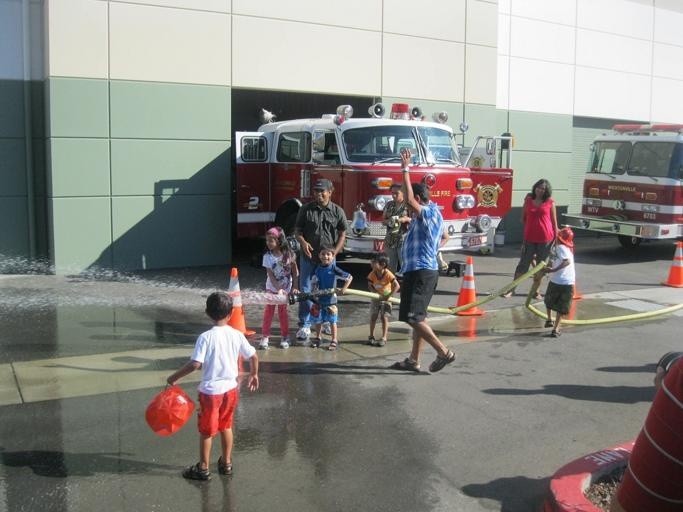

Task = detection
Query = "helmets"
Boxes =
[146,385,194,436]
[557,226,574,248]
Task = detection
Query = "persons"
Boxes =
[259,227,300,350]
[500,179,559,299]
[388,147,455,372]
[365,253,400,347]
[294,178,348,339]
[309,243,353,351]
[541,227,576,337]
[166,293,260,481]
[380,182,412,278]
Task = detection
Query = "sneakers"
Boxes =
[378,338,387,345]
[368,336,375,345]
[259,322,338,350]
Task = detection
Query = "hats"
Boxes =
[312,179,332,191]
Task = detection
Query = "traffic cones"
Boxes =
[455,314,478,341]
[450,255,489,319]
[566,299,576,323]
[662,242,682,291]
[225,263,254,338]
[571,265,581,301]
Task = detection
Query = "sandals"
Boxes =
[545,321,554,327]
[430,350,455,371]
[183,463,212,481]
[218,456,233,475]
[551,330,561,337]
[390,358,420,371]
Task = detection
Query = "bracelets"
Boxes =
[402,166,409,172]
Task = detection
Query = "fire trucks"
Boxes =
[558,121,682,254]
[233,101,517,278]
